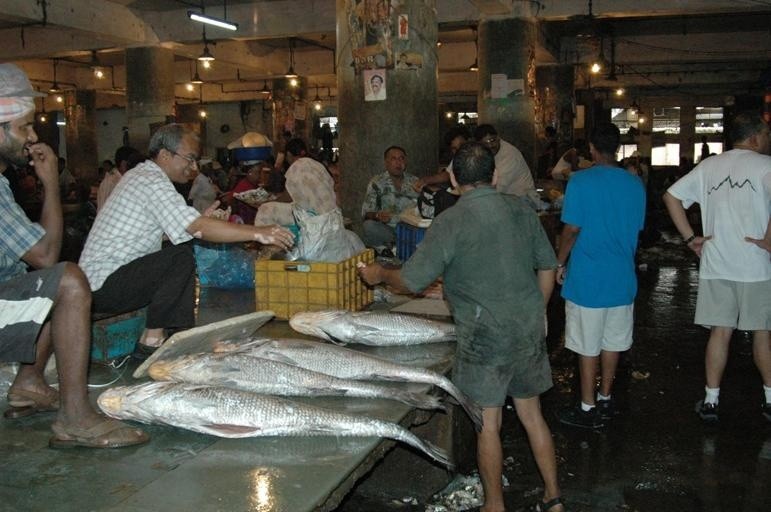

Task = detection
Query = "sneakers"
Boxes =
[695,400,719,421]
[557,399,609,429]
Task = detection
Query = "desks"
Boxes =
[536,207,562,249]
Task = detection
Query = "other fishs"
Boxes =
[147,351,449,414]
[96,381,456,471]
[289,309,458,348]
[159,435,384,462]
[356,343,456,368]
[211,335,486,434]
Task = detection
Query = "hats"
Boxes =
[0,61,47,98]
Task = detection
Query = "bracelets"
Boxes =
[682,234,697,245]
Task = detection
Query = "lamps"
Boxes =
[469,22,479,72]
[186,0,323,118]
[36,56,114,124]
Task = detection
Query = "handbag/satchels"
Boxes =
[416,180,462,219]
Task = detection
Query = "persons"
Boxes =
[663,110,771,422]
[1,124,338,369]
[400,16,407,37]
[1,63,151,449]
[360,122,649,429]
[397,54,409,68]
[358,141,564,511]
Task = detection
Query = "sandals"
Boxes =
[4,386,59,419]
[49,421,151,450]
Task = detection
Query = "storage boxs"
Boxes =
[253,248,375,321]
[393,221,427,265]
